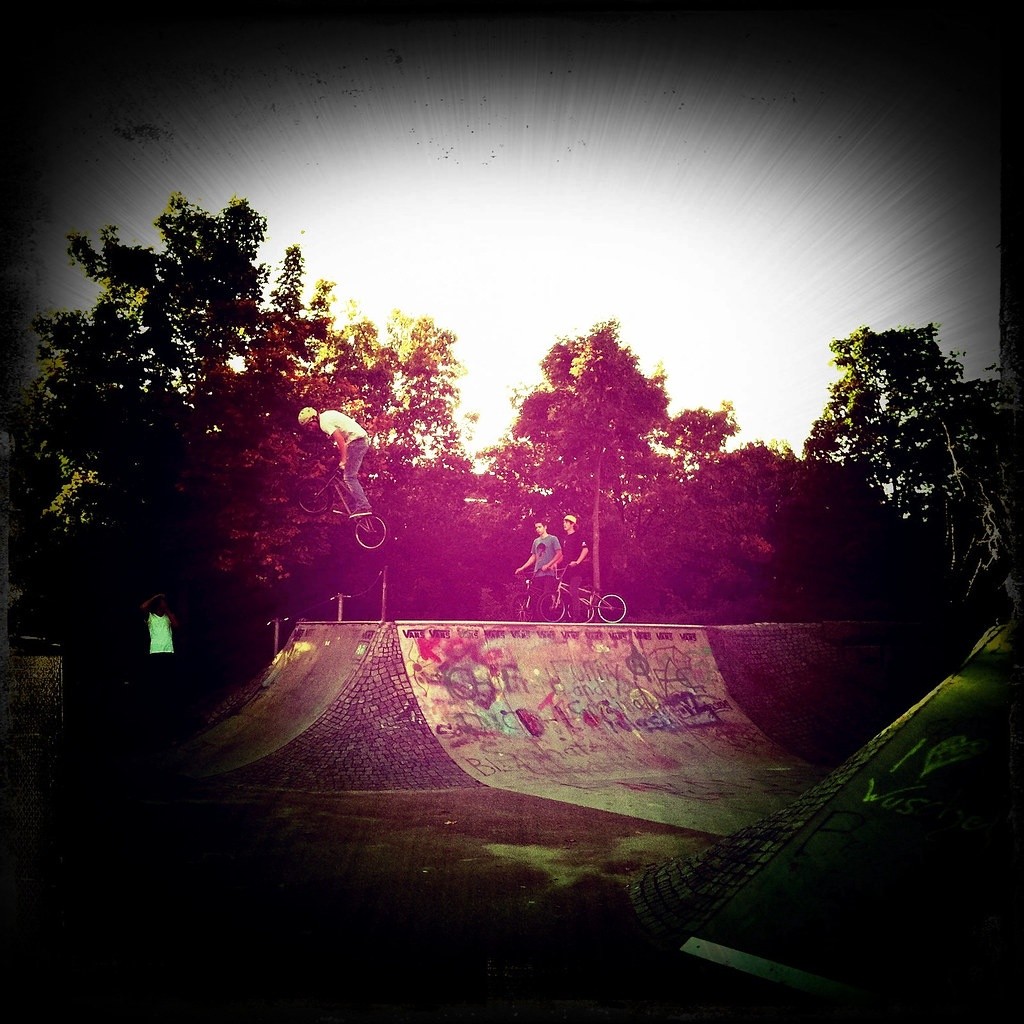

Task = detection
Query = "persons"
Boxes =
[515,515,589,623]
[298,407,373,519]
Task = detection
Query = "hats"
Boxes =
[565,515,578,524]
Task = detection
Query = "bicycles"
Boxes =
[299,466,389,552]
[512,564,627,624]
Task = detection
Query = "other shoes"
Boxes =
[349,510,373,518]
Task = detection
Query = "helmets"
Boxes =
[298,406,317,426]
